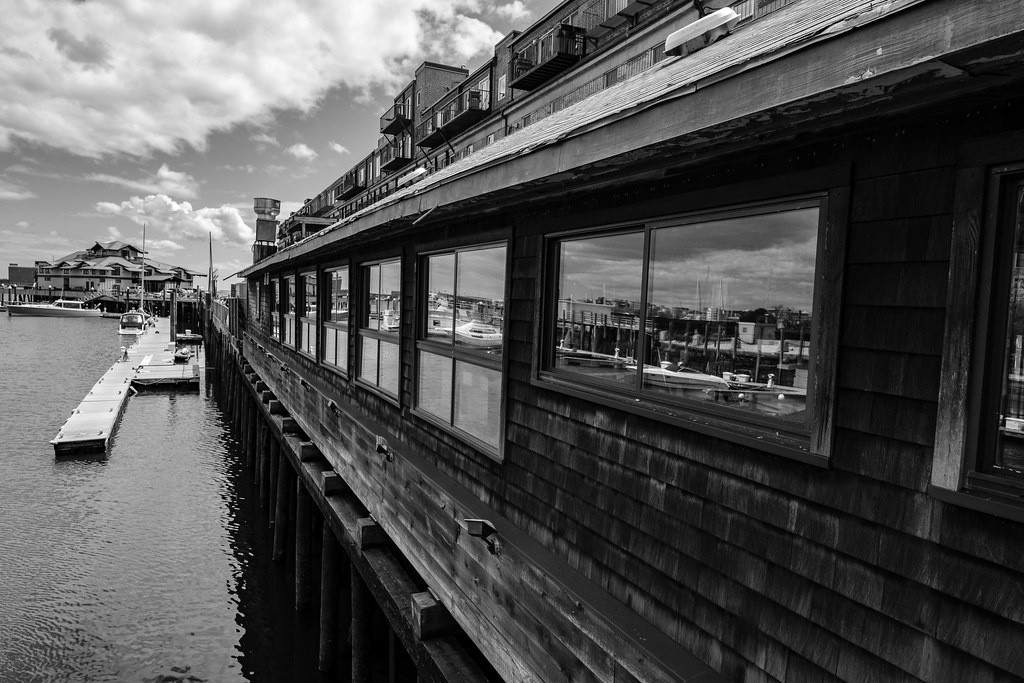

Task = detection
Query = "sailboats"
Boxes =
[118,223,153,337]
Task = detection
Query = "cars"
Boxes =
[109,283,196,300]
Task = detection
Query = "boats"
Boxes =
[550,331,808,400]
[6,299,105,319]
[298,285,503,351]
